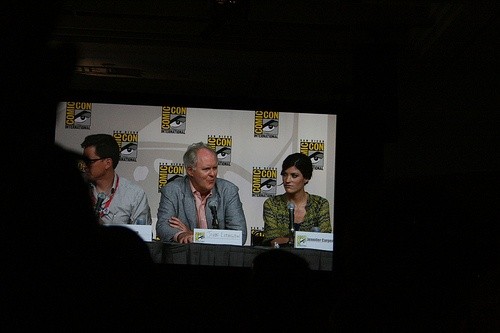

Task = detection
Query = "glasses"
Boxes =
[84,159,101,164]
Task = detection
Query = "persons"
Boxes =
[231,249,324,332]
[79,133,152,225]
[156,142,247,246]
[262,152,331,248]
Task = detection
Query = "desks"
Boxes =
[146,241,332,270]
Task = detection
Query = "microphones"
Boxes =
[95,191,106,217]
[287,201,295,233]
[209,200,219,224]
